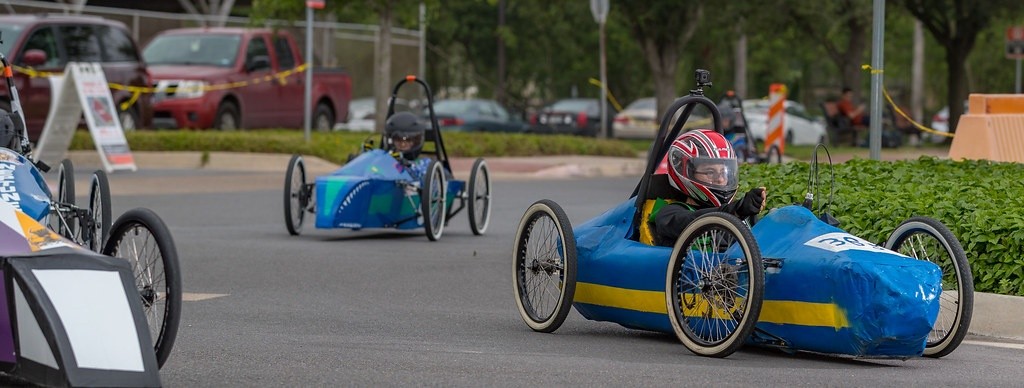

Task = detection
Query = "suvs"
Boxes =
[0,12,156,136]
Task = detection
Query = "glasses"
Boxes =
[693,169,730,179]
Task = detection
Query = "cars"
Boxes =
[612,96,694,139]
[931,100,970,140]
[719,95,831,148]
[529,96,619,137]
[414,97,536,135]
[331,94,425,135]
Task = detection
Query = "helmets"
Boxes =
[383,111,425,160]
[667,129,739,208]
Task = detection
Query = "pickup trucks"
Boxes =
[136,26,353,132]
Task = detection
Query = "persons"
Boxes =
[838,87,870,127]
[382,111,433,176]
[655,129,767,253]
